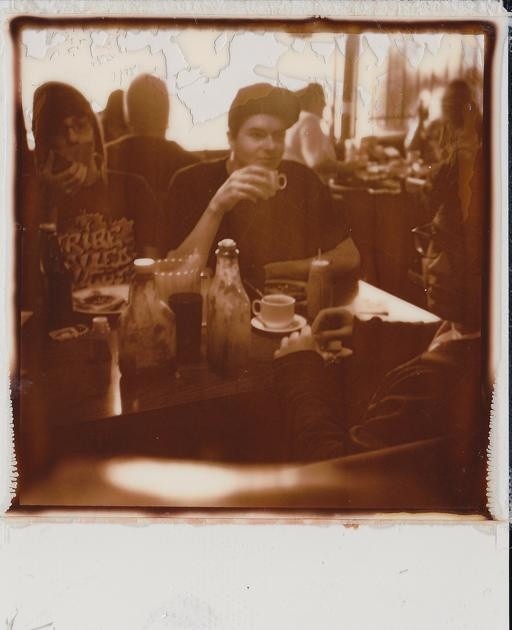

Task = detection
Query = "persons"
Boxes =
[111,75,207,226]
[272,144,483,463]
[18,79,161,311]
[407,77,480,162]
[91,88,130,145]
[280,81,370,180]
[153,82,360,279]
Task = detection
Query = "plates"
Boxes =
[262,278,309,306]
[250,314,307,335]
[71,283,132,314]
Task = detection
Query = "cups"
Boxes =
[252,294,297,330]
[256,169,287,199]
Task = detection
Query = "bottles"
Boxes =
[37,223,75,324]
[307,260,333,321]
[207,237,252,378]
[89,315,113,390]
[118,257,177,379]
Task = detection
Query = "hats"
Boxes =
[228,83,301,132]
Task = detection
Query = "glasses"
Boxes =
[410,222,443,259]
[53,121,92,137]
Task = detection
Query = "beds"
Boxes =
[48,274,443,428]
